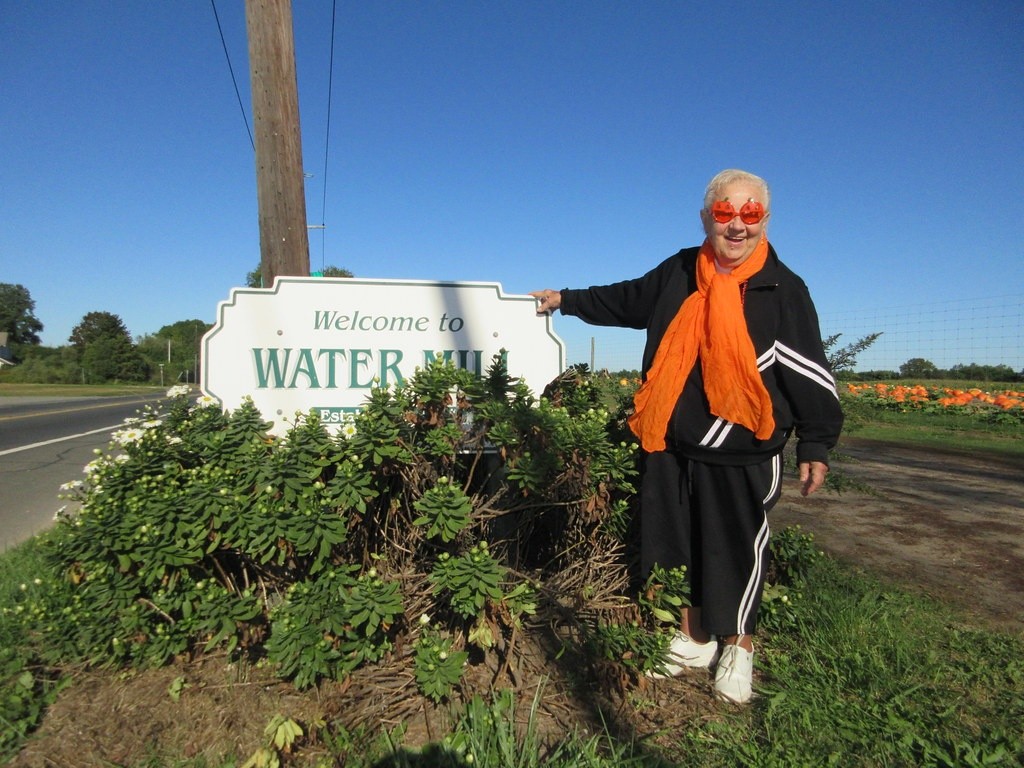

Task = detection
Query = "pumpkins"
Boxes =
[846,383,1024,411]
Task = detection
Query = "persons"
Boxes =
[530,169,845,702]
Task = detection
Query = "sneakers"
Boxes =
[645,630,722,678]
[714,634,754,702]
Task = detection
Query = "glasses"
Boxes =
[706,197,764,224]
